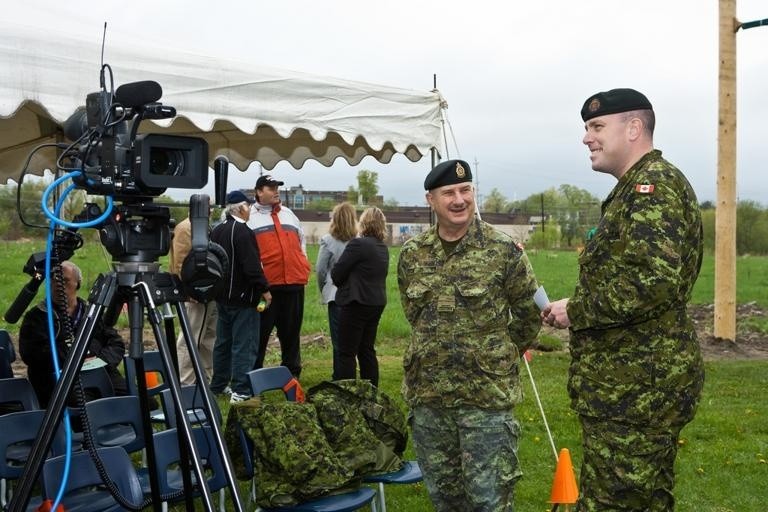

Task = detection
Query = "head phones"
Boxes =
[180,193,233,295]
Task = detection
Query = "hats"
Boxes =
[226,190,255,204]
[424,160,472,191]
[256,176,284,189]
[581,87,653,122]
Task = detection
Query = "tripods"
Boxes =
[4,260,256,512]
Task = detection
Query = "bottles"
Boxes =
[257,295,269,311]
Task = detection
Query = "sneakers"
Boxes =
[223,387,232,394]
[228,392,252,404]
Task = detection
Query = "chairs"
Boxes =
[0,378,40,414]
[124,352,170,398]
[41,446,142,512]
[71,368,115,406]
[233,402,377,512]
[0,330,17,362]
[1,411,66,508]
[149,383,223,429]
[67,396,146,453]
[246,366,422,512]
[0,346,14,379]
[134,426,228,511]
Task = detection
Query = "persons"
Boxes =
[330,207,390,389]
[539,88,707,512]
[397,161,542,512]
[209,191,273,406]
[169,194,219,388]
[247,175,310,381]
[316,202,361,381]
[18,258,159,450]
